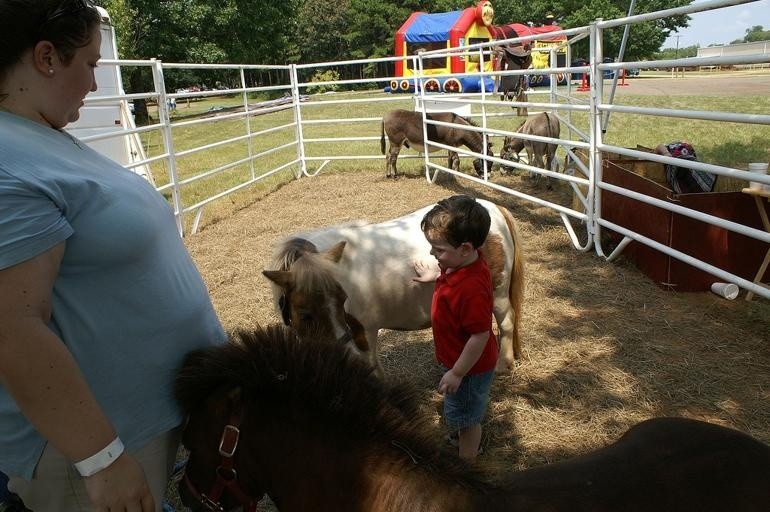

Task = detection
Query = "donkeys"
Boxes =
[378,108,495,180]
[162,315,770,512]
[497,111,562,190]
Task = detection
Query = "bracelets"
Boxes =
[74,436,126,478]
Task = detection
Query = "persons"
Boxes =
[655,142,718,195]
[411,195,500,465]
[166,97,171,111]
[0,0,228,512]
[170,97,176,111]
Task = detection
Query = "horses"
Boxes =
[260,194,531,383]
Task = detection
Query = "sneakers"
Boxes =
[448,430,483,453]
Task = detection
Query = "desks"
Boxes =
[741,187,770,300]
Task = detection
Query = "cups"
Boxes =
[747,162,769,191]
[710,282,741,301]
